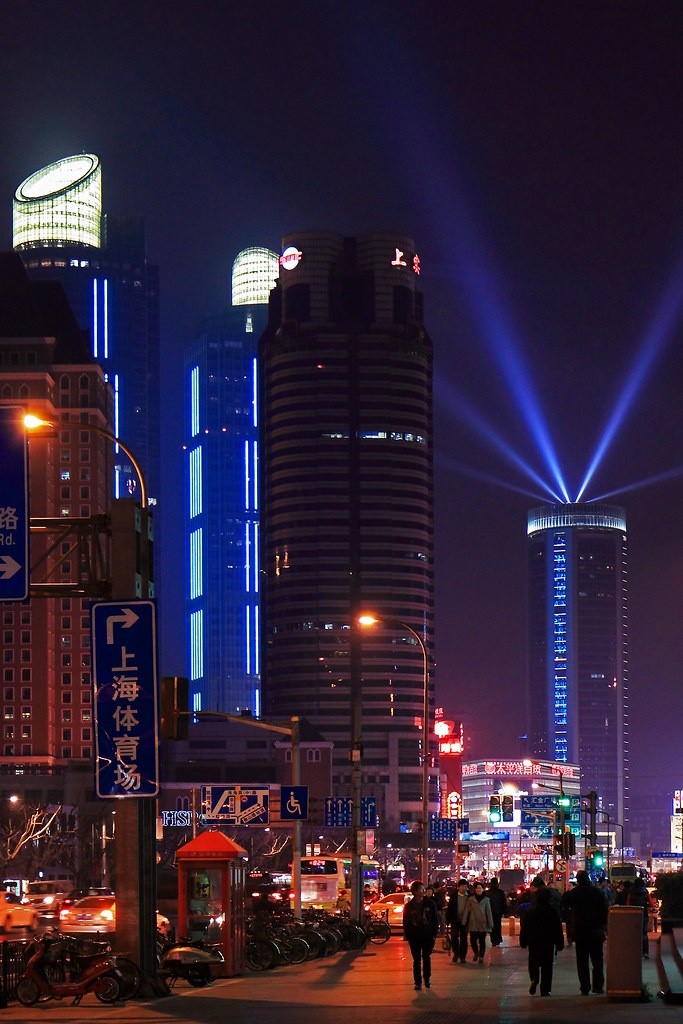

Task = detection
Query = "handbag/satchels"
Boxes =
[442,925,451,950]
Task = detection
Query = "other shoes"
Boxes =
[529,978,538,995]
[478,958,484,964]
[591,989,605,994]
[581,991,589,996]
[473,953,479,961]
[424,978,430,988]
[541,992,551,997]
[644,953,649,959]
[453,954,459,962]
[460,959,468,965]
[414,982,422,990]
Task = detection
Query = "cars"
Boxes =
[0,877,169,938]
[371,893,414,927]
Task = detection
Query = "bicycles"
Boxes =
[247,907,391,971]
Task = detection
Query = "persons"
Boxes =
[562,870,611,996]
[638,867,649,878]
[364,884,377,899]
[507,886,518,912]
[423,887,438,909]
[336,888,351,911]
[445,879,493,963]
[486,877,509,946]
[596,877,655,959]
[518,877,564,996]
[396,885,409,893]
[403,882,439,990]
[659,869,664,873]
[433,881,451,950]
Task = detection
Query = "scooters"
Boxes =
[16,924,225,1007]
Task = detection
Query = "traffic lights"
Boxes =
[503,795,513,822]
[554,834,567,859]
[560,795,571,808]
[592,851,603,867]
[488,795,501,824]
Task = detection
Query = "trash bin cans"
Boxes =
[605,906,645,1001]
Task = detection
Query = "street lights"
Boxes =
[359,612,430,890]
[601,821,624,870]
[24,414,160,999]
[523,760,568,895]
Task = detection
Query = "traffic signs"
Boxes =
[88,601,161,800]
[520,794,583,840]
[0,404,30,605]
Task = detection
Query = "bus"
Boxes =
[289,857,382,913]
[609,863,641,886]
